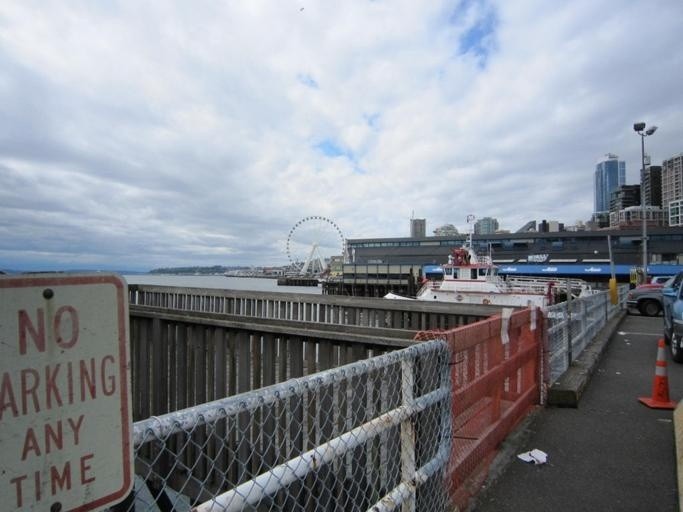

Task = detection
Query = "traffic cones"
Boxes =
[636,338,678,409]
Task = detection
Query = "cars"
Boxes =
[625,271,683,363]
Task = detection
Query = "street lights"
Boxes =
[633,122,658,283]
